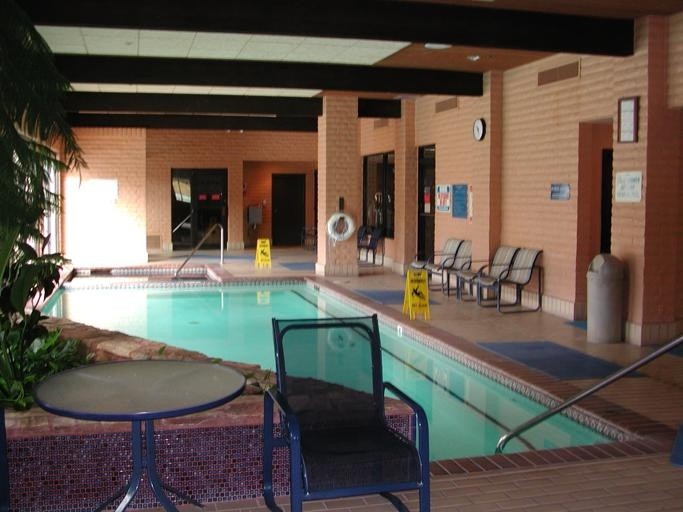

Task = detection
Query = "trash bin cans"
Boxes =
[586,253,626,344]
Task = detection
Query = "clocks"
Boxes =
[472,118,485,142]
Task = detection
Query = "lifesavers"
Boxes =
[327,213,355,240]
[327,327,353,352]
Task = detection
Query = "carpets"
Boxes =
[190,254,254,262]
[279,262,315,272]
[475,338,649,383]
[354,288,440,306]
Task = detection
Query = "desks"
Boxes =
[33,358,247,511]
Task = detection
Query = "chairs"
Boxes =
[261,313,431,511]
[356,223,385,265]
[410,238,544,314]
